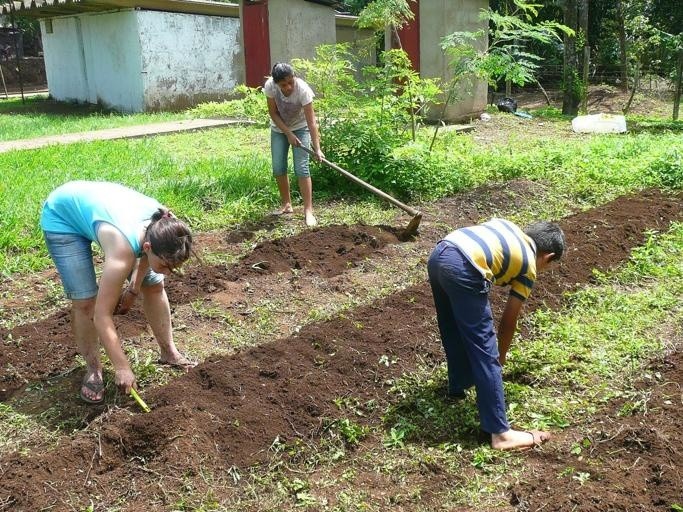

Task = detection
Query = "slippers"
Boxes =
[490,430,551,452]
[157,357,198,369]
[82,366,105,404]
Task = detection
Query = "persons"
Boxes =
[40,180,197,404]
[263,61,325,226]
[426,217,566,453]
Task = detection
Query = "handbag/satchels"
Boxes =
[498,95,517,114]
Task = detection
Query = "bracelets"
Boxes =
[126,289,138,297]
